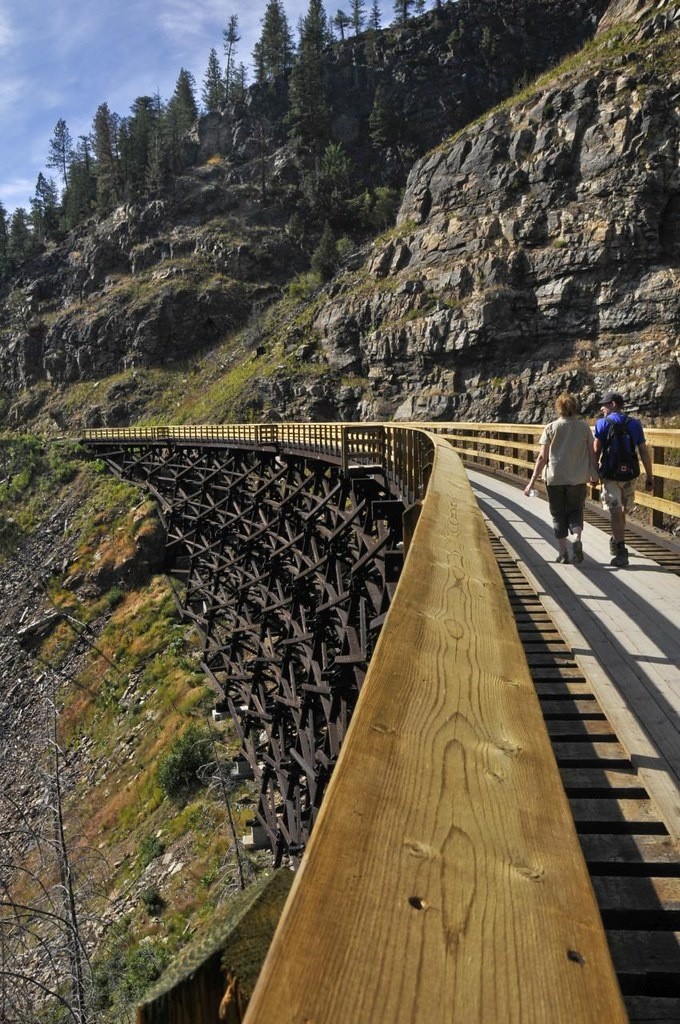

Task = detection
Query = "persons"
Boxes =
[589,392,654,565]
[525,396,599,564]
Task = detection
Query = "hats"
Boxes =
[598,392,624,407]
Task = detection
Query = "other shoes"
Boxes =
[609,535,617,556]
[556,550,569,564]
[569,538,584,564]
[610,544,628,566]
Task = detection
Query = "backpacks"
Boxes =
[598,416,640,482]
